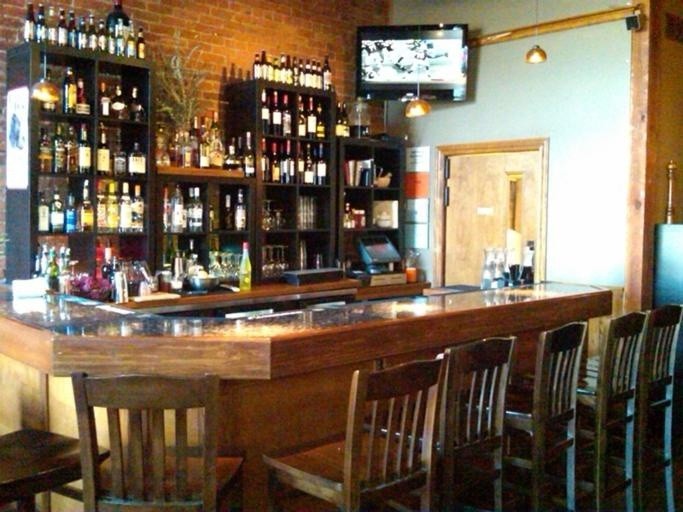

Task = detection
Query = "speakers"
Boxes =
[625,10,645,31]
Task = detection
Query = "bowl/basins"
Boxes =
[183,276,220,293]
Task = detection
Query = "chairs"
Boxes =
[69,369,247,511]
[0,427,109,510]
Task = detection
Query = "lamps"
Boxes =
[403,1,430,119]
[523,0,546,66]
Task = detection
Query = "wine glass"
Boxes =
[207,251,243,284]
[262,243,291,281]
[260,197,286,231]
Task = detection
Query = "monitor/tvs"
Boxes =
[355,24,468,102]
[353,233,402,271]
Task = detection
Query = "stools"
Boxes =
[259,352,450,510]
[512,310,645,508]
[364,334,517,510]
[579,303,681,480]
[459,320,589,510]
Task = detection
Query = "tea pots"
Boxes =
[480,245,523,292]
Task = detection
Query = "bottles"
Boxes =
[39,122,89,174]
[50,318,203,338]
[35,179,93,235]
[93,237,196,305]
[182,111,256,178]
[239,241,252,293]
[96,181,144,234]
[40,294,71,323]
[159,184,204,232]
[96,127,147,176]
[260,137,327,185]
[41,66,92,115]
[99,82,147,122]
[405,251,417,283]
[208,186,248,233]
[351,96,371,138]
[250,48,333,91]
[334,101,350,138]
[261,87,328,140]
[32,244,74,293]
[342,203,355,229]
[22,0,148,59]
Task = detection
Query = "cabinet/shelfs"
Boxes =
[334,131,404,266]
[159,163,255,268]
[3,39,157,279]
[228,78,336,270]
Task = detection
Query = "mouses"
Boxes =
[367,265,381,274]
